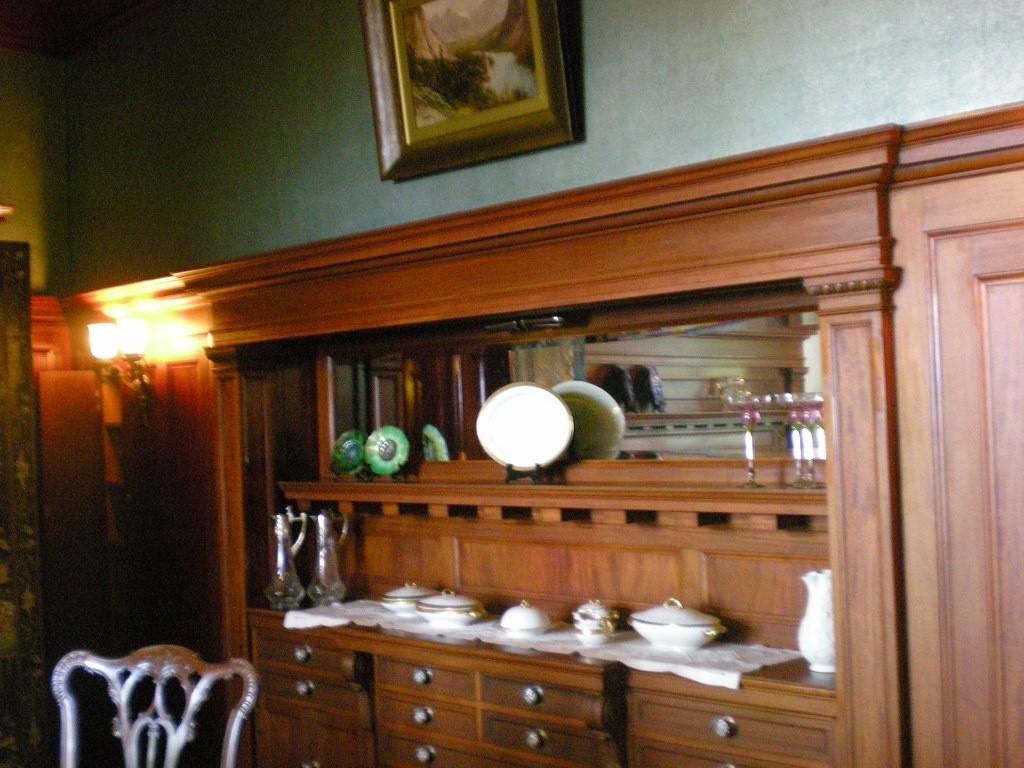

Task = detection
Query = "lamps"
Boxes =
[84,314,199,546]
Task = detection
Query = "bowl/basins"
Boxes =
[384,584,728,651]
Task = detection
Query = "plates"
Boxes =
[331,424,412,475]
[550,381,626,461]
[474,382,575,473]
[422,424,450,462]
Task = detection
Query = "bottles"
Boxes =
[796,569,836,674]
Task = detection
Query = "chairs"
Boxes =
[50,645,260,768]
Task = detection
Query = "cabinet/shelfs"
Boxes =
[244,609,840,767]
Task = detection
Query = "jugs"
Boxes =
[306,508,353,608]
[260,511,308,609]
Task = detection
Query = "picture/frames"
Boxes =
[358,0,579,182]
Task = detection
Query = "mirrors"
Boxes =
[332,306,824,466]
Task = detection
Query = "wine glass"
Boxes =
[723,391,827,490]
